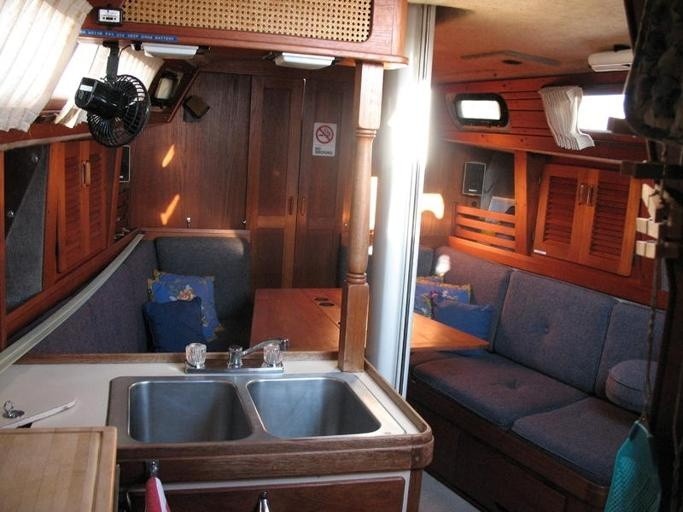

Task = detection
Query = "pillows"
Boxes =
[417,275,444,283]
[148,276,217,344]
[432,298,495,358]
[153,269,224,334]
[251,286,493,374]
[144,297,207,355]
[414,280,471,321]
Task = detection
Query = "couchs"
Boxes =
[26,229,256,355]
[342,245,668,512]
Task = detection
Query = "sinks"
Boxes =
[245,370,407,440]
[106,374,257,446]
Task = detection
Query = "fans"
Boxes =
[75,40,152,148]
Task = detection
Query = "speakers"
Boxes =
[461,161,487,196]
[119,145,131,183]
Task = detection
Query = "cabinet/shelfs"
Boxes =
[246,57,358,292]
[0,426,120,512]
[529,156,644,274]
[56,138,121,282]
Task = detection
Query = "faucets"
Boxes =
[225,337,289,369]
